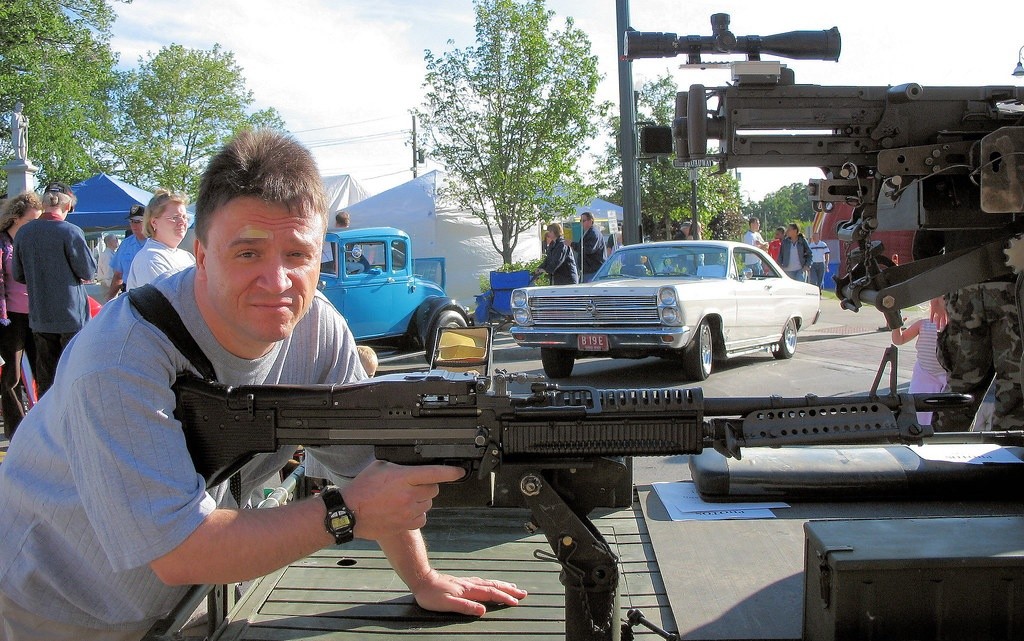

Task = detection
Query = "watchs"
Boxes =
[318,488,356,546]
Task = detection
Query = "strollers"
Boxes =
[466,270,544,336]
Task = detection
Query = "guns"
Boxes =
[167,326,975,641]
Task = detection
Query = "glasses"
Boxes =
[154,214,190,225]
[68,206,74,213]
[130,219,144,223]
[580,219,589,223]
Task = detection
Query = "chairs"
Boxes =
[620,253,646,276]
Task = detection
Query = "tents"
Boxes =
[177,173,374,265]
[531,182,623,221]
[64,172,195,230]
[321,168,573,307]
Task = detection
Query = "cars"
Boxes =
[315,226,471,357]
[509,240,822,380]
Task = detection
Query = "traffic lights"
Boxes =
[641,124,674,157]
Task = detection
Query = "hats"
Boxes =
[125,206,144,219]
[45,182,74,200]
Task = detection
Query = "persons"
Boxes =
[331,212,350,261]
[0,128,527,641]
[808,232,831,300]
[768,227,786,263]
[99,234,119,289]
[743,217,769,276]
[536,222,579,285]
[930,282,1024,431]
[674,221,704,276]
[887,318,948,426]
[606,223,623,257]
[104,204,150,301]
[0,190,47,445]
[12,183,99,402]
[126,188,196,294]
[776,223,813,282]
[542,232,550,256]
[565,213,606,283]
